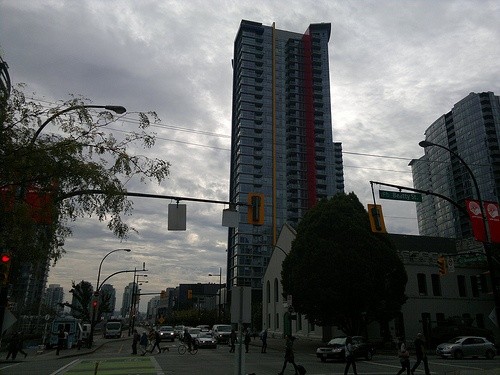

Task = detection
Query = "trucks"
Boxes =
[79,323,91,344]
[104,319,123,339]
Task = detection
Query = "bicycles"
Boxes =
[177,341,199,355]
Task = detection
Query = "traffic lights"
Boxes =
[92,298,98,309]
[0,252,12,289]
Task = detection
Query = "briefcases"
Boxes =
[297,365,306,375]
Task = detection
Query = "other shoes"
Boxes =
[411,369,415,375]
[187,348,192,352]
[245,351,248,353]
[229,350,235,352]
[132,352,137,354]
[261,351,267,353]
[141,352,146,356]
[278,372,284,375]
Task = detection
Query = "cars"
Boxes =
[316,335,377,363]
[435,335,496,361]
[134,319,156,327]
[176,325,233,349]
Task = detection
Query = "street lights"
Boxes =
[89,249,131,347]
[419,140,500,326]
[132,274,148,335]
[128,267,148,337]
[133,275,149,333]
[208,267,222,324]
[18,105,127,202]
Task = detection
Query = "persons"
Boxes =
[396,337,410,375]
[149,331,161,353]
[410,333,432,375]
[244,330,251,353]
[131,329,138,354]
[261,330,268,353]
[77,330,83,350]
[344,337,359,375]
[56,326,69,356]
[229,329,237,353]
[6,328,28,360]
[180,332,192,352]
[278,334,299,375]
[139,330,147,356]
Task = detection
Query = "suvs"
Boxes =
[156,325,176,342]
